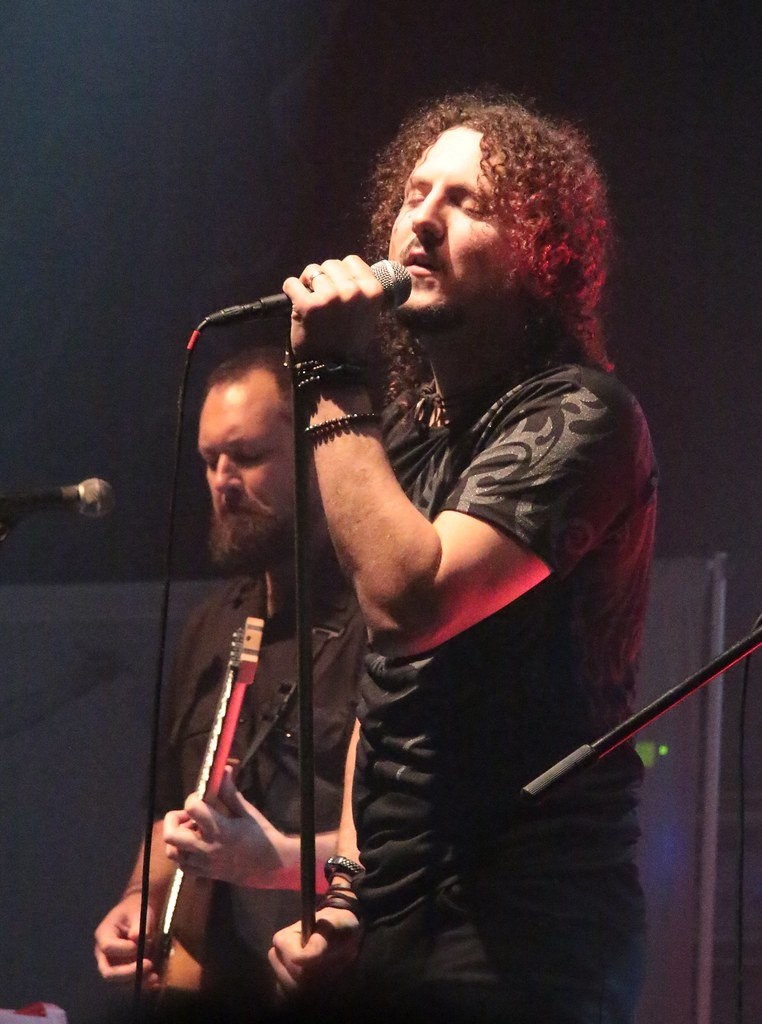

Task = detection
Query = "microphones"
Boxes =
[204,258,413,326]
[0,476,115,523]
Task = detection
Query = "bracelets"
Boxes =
[292,351,380,439]
[315,856,364,919]
[120,883,142,902]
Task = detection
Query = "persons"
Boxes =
[94,344,367,1023]
[268,94,656,1024]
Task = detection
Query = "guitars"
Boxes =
[98,616,268,1024]
